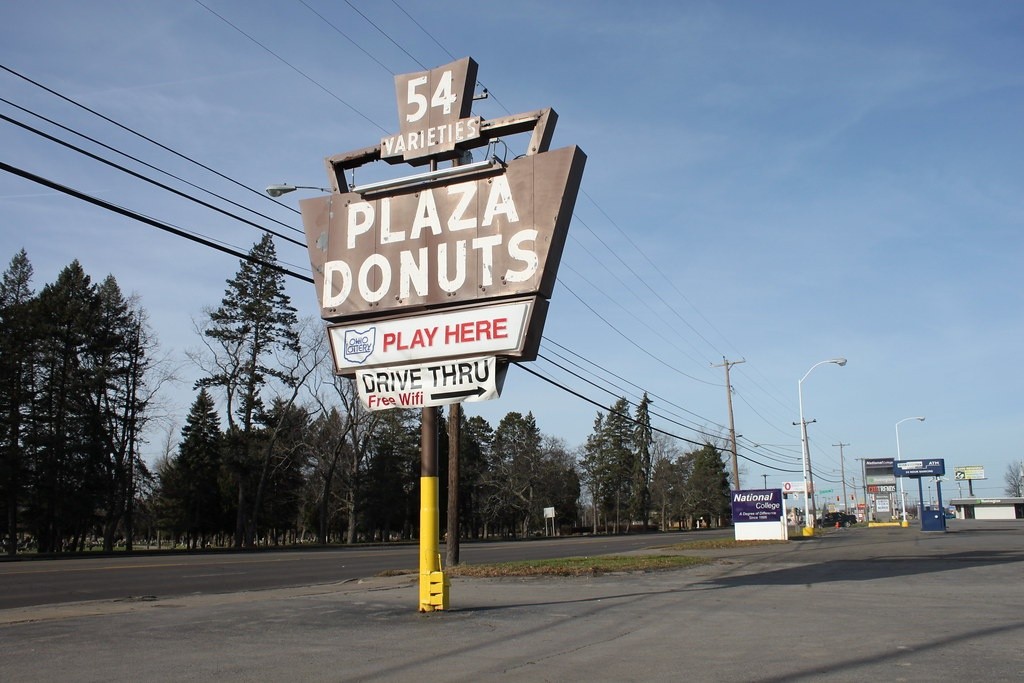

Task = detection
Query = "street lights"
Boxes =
[792,358,845,537]
[266,182,333,208]
[894,415,926,528]
